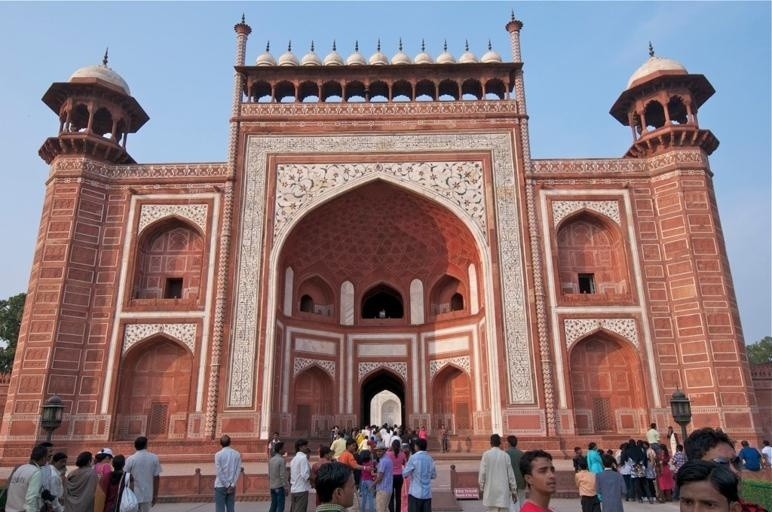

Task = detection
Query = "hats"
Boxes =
[373,443,388,450]
[97,448,114,458]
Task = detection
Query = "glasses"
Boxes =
[710,456,741,467]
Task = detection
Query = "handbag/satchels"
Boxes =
[119,472,139,512]
[93,482,107,512]
[0,488,9,512]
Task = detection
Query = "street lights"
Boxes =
[669,383,695,445]
[39,391,67,444]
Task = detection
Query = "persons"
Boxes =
[0,436,162,512]
[504,435,526,512]
[478,434,518,512]
[518,449,556,512]
[214,434,241,511]
[573,423,772,512]
[440,424,448,453]
[268,423,436,512]
